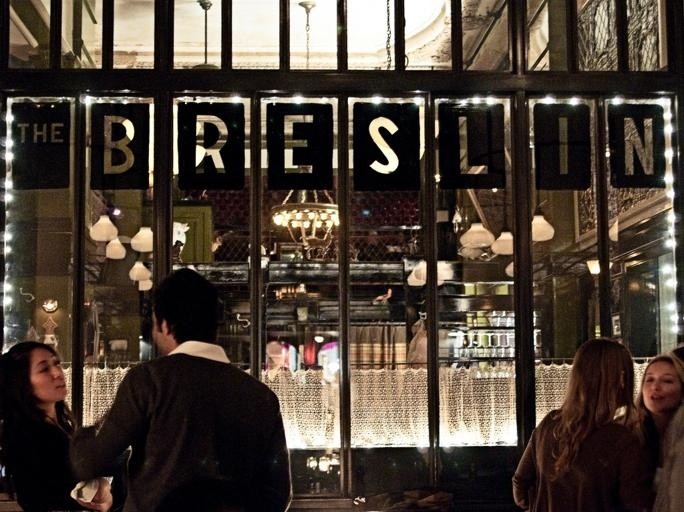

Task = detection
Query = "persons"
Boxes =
[510,337,652,512]
[616,354,684,442]
[652,399,684,512]
[0,341,124,512]
[67,268,294,512]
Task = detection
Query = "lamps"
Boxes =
[271,2,340,249]
[458,190,555,279]
[89,191,154,291]
